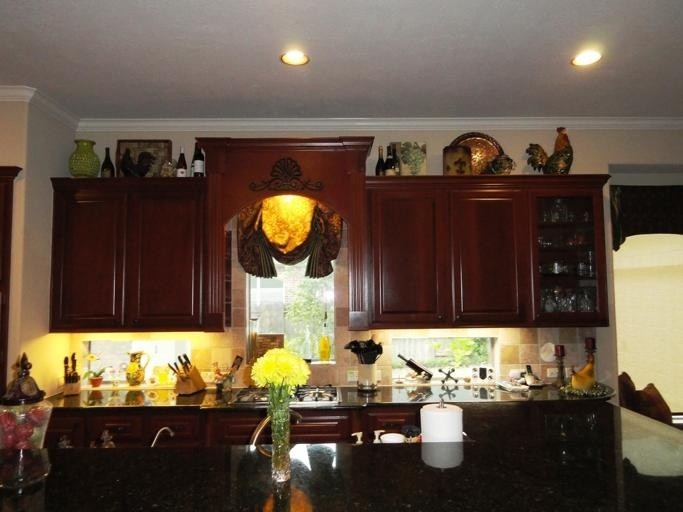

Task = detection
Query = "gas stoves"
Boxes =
[229,382,342,413]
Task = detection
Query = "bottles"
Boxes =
[396,354,433,380]
[374,144,399,176]
[100,147,114,176]
[493,155,512,175]
[542,287,595,313]
[175,142,204,177]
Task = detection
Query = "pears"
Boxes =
[571,361,596,392]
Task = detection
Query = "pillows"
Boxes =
[627,382,672,426]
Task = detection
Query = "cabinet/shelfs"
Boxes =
[350,406,418,448]
[0,166,23,397]
[49,176,225,332]
[205,408,349,447]
[89,408,146,447]
[146,406,205,448]
[43,407,88,448]
[530,189,609,327]
[372,191,530,329]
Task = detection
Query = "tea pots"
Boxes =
[127,352,149,385]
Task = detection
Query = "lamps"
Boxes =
[237,194,344,279]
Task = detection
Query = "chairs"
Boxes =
[618,371,683,431]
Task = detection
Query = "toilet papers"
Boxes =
[420,404,463,442]
[420,443,465,468]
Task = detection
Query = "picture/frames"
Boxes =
[117,139,172,178]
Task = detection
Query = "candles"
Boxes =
[420,399,463,470]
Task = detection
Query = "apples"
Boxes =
[0,448,47,485]
[1,407,46,448]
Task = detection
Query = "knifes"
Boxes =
[168,355,193,374]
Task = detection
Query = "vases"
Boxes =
[88,376,104,387]
[270,404,290,483]
[215,383,224,401]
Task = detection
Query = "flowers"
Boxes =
[250,347,312,409]
[212,362,233,384]
[82,354,108,378]
